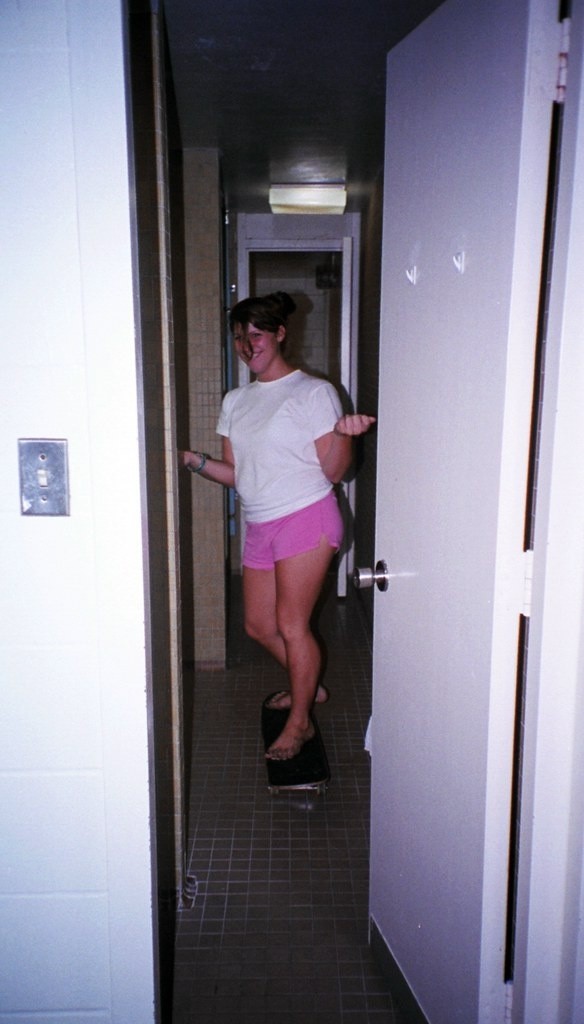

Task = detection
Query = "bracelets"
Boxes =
[187,452,206,472]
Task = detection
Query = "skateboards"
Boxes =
[260,689,332,796]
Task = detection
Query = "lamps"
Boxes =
[268,183,348,215]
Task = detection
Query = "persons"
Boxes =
[177,292,376,761]
